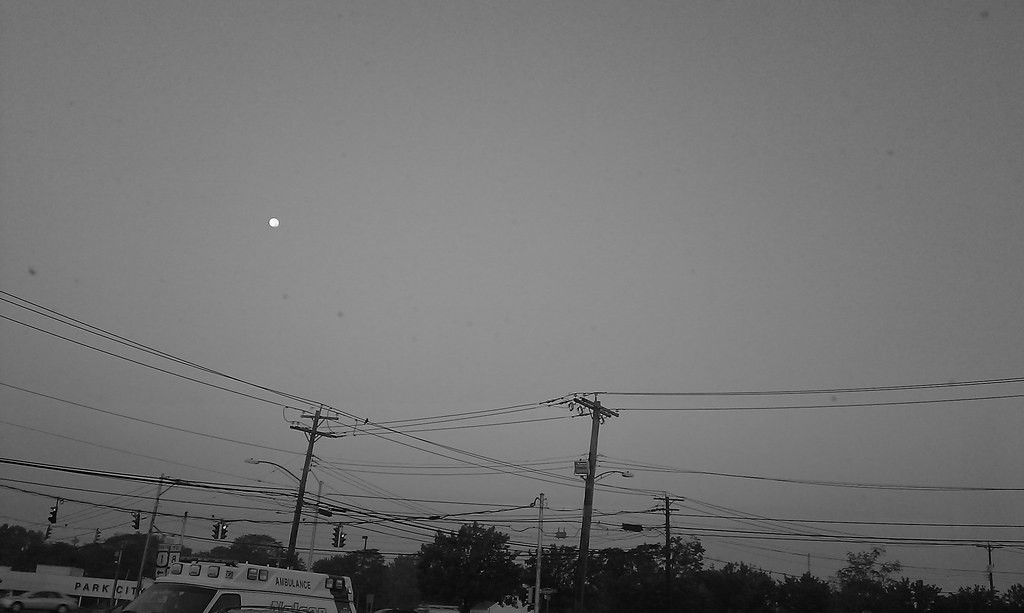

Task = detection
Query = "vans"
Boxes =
[122,558,356,613]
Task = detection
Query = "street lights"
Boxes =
[245,410,339,569]
[572,397,635,611]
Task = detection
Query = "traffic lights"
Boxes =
[211,524,219,540]
[332,527,338,547]
[339,532,346,548]
[220,524,228,539]
[131,512,140,529]
[48,505,58,524]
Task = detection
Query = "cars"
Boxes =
[0,588,79,613]
